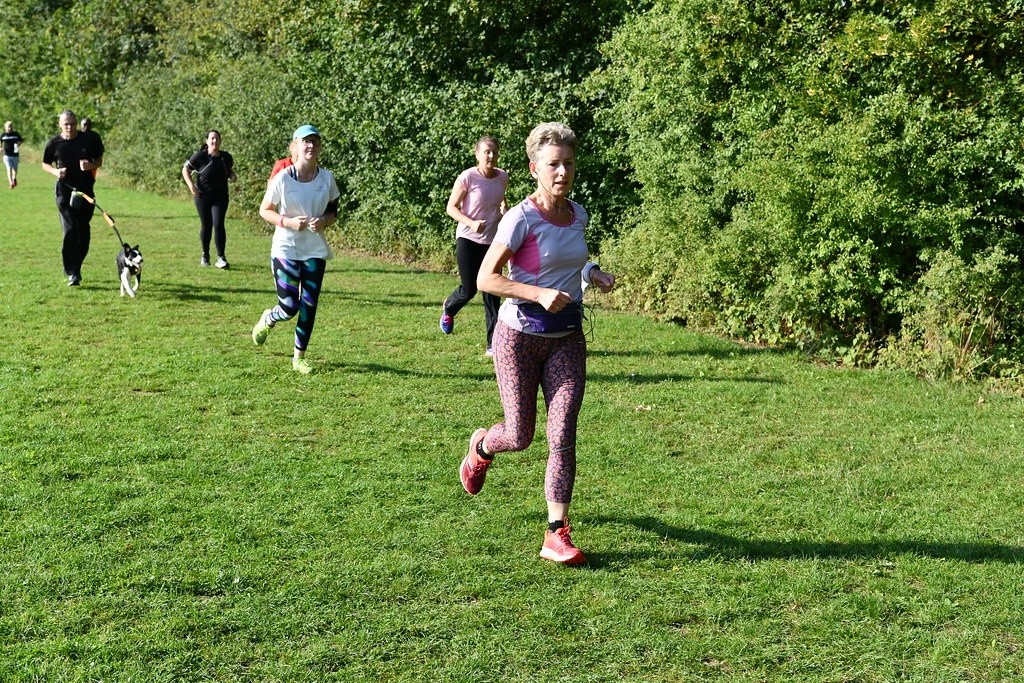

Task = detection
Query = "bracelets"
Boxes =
[582,262,599,284]
[277,216,286,229]
[323,214,332,225]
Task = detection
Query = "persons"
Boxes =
[461,121,617,563]
[252,125,340,374]
[78,117,105,178]
[439,136,508,357]
[0,121,24,189]
[42,110,103,287]
[181,130,237,269]
[267,142,296,183]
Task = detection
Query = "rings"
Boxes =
[554,306,559,310]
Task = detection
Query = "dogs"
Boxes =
[117,243,143,298]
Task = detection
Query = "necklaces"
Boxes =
[296,165,316,183]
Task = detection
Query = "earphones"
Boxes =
[534,171,538,175]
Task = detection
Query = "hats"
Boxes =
[293,126,322,142]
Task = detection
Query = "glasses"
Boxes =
[298,139,321,145]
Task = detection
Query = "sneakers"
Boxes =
[486,349,492,355]
[201,254,210,266]
[440,299,455,334]
[292,358,314,373]
[540,517,586,564]
[68,275,80,285]
[460,427,493,495]
[213,259,229,268]
[252,309,272,346]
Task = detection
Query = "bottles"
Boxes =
[13,143,19,153]
